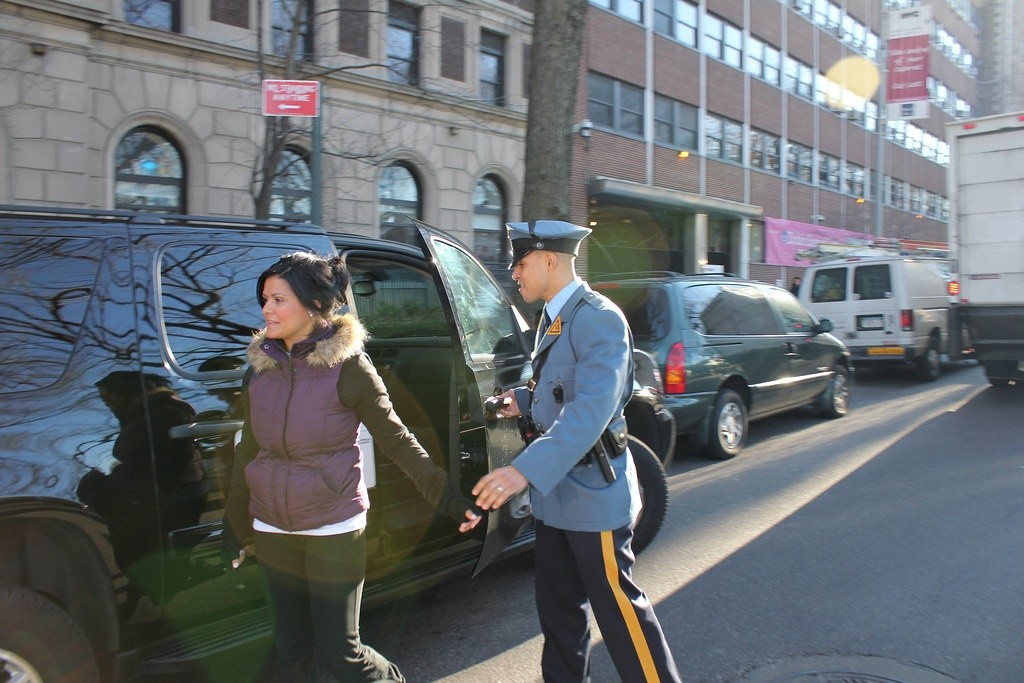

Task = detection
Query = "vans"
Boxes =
[796,249,969,384]
[0,203,669,683]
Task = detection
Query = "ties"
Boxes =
[536,307,552,348]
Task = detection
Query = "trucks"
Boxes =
[945,109,1023,393]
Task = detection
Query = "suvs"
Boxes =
[588,266,853,461]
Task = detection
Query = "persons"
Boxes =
[200,355,252,419]
[790,277,802,297]
[471,219,687,683]
[219,252,486,683]
[77,369,269,630]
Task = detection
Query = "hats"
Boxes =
[505,220,592,271]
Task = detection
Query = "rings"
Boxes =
[497,487,503,492]
